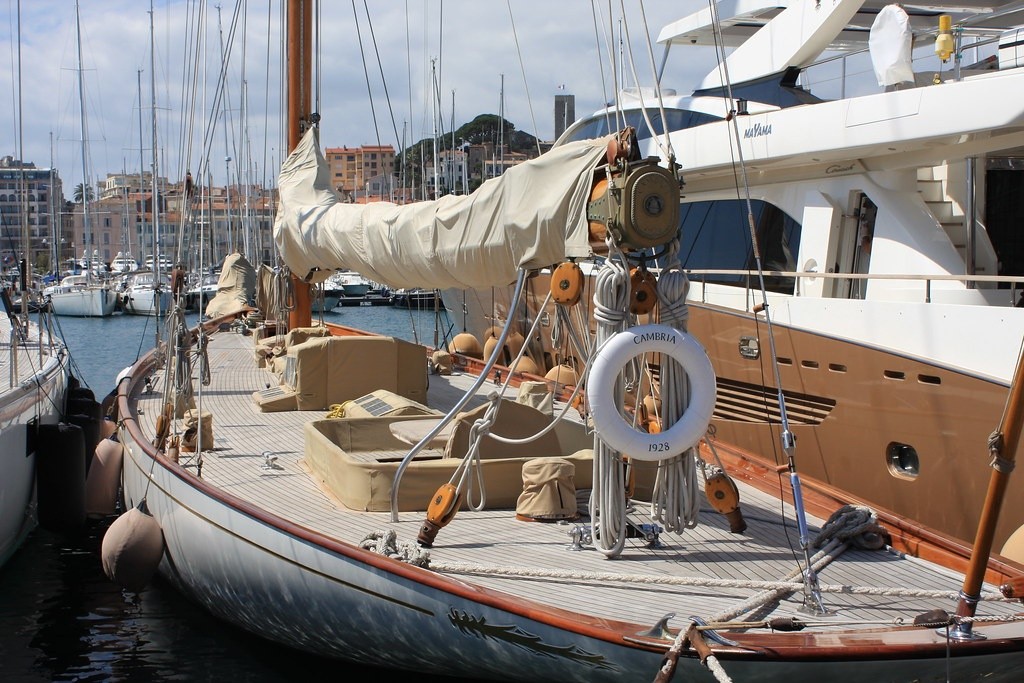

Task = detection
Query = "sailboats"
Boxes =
[231,2,648,310]
[38,0,222,318]
[87,3,1024,681]
[0,0,95,573]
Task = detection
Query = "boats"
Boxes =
[110,252,138,272]
[75,249,106,271]
[435,0,1024,683]
[141,255,172,270]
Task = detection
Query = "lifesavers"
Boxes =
[581,320,722,467]
